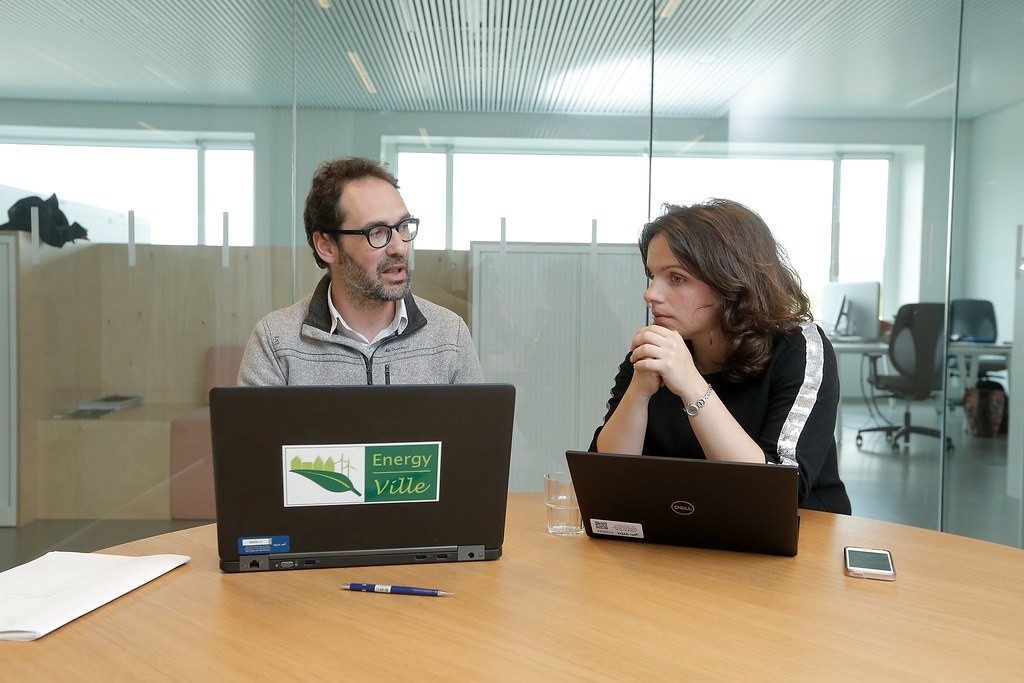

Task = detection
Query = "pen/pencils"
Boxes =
[340,582,457,597]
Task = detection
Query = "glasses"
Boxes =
[320,215,420,249]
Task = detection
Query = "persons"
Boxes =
[237,157,486,387]
[589,197,852,517]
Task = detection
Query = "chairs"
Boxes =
[858,305,956,452]
[947,299,1013,411]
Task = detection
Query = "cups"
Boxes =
[544,472,585,536]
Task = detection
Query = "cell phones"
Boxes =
[844,546,896,581]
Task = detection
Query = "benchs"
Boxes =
[172,341,251,523]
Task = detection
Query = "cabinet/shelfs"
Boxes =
[468,240,664,493]
[39,403,209,521]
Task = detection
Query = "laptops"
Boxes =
[564,451,802,559]
[209,384,516,573]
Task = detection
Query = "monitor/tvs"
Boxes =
[813,282,881,342]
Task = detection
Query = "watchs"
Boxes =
[684,382,712,416]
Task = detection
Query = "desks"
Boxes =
[0,492,1024,683]
[831,341,1013,444]
